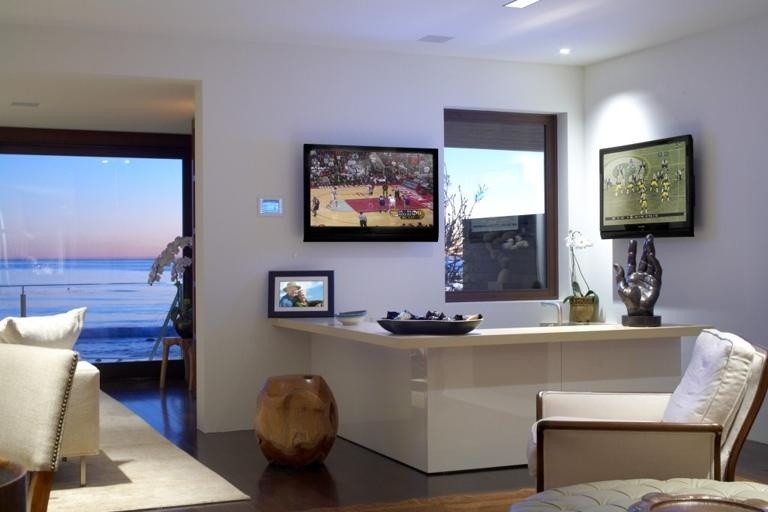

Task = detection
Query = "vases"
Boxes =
[171,314,194,340]
[568,293,599,322]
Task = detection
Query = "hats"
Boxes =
[282,282,301,292]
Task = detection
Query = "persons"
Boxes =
[279,282,301,307]
[358,212,366,227]
[327,187,339,207]
[387,193,396,215]
[310,150,432,195]
[612,233,662,315]
[294,288,323,307]
[311,197,319,216]
[603,157,681,215]
[377,195,386,213]
[399,193,411,210]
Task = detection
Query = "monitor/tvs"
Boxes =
[304,142,439,242]
[599,135,695,239]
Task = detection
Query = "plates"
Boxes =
[375,314,483,336]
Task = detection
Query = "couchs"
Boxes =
[53,359,102,488]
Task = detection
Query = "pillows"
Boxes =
[1,307,87,352]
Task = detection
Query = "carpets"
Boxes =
[43,384,254,512]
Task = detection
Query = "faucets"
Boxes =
[539,299,563,324]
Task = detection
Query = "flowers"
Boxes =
[146,230,193,325]
[562,227,599,306]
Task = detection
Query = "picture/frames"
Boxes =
[267,268,335,319]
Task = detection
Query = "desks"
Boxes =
[503,471,768,511]
[263,311,716,477]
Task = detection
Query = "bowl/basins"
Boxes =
[334,310,368,326]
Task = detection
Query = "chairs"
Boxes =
[521,327,767,494]
[0,343,79,511]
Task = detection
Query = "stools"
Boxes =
[251,370,341,472]
[158,334,196,394]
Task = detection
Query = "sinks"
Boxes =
[535,320,583,326]
